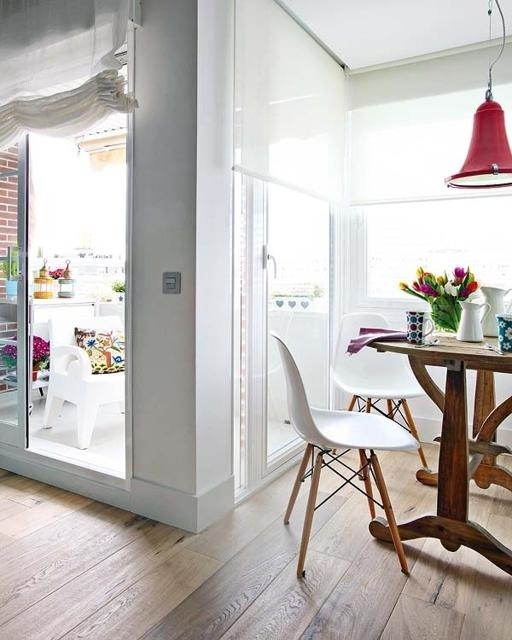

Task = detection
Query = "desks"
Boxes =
[365,332,512,576]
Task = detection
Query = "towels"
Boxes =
[347,327,408,355]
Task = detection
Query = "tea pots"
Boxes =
[455,300,491,342]
[478,286,512,338]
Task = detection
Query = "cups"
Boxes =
[496,314,512,354]
[405,309,435,347]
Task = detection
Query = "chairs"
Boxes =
[271,334,420,578]
[43,300,125,450]
[332,315,432,479]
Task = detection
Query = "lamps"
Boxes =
[443,2,512,190]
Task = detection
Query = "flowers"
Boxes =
[398,267,480,307]
[1,334,49,371]
[48,268,66,280]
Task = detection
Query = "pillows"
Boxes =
[75,327,124,374]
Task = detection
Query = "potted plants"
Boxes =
[1,240,20,300]
[112,280,125,301]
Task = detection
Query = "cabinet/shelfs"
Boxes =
[32,302,126,394]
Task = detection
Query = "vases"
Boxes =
[427,301,464,336]
[32,371,38,381]
